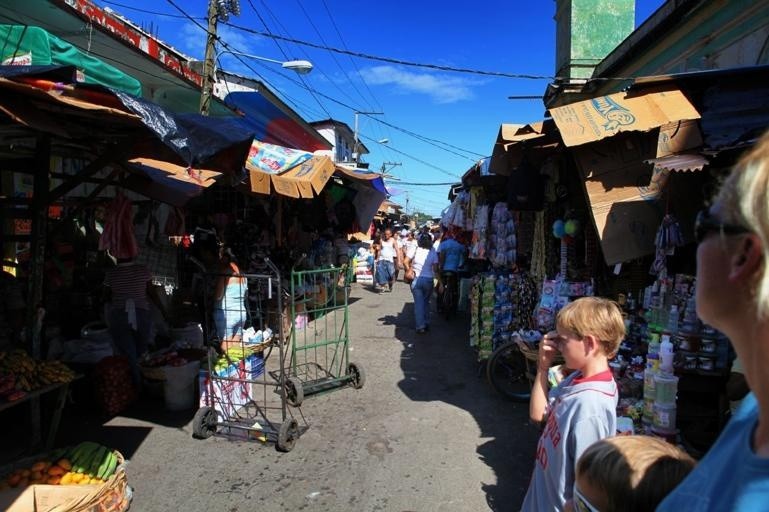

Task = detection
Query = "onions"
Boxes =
[165,351,188,366]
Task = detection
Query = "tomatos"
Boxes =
[8,457,70,488]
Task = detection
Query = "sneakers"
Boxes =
[388,284,394,292]
[380,286,386,294]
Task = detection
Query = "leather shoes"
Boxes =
[418,331,425,336]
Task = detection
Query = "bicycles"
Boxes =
[435,264,463,319]
[487,334,544,404]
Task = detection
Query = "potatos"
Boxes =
[96,360,135,418]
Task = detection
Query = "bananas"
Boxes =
[1,345,73,393]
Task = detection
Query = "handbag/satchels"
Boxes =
[404,268,415,280]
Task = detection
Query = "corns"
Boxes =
[67,441,118,482]
[203,346,254,371]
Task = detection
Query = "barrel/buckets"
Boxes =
[138,344,207,412]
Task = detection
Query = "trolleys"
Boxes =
[268,250,366,407]
[187,256,299,452]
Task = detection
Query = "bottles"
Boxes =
[240,327,273,347]
[669,305,679,331]
[627,292,633,308]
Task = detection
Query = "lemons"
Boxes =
[59,470,106,490]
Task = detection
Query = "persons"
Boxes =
[211,243,247,353]
[373,224,466,336]
[656,132,769,512]
[521,297,696,512]
[101,254,165,395]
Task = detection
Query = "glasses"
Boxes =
[569,480,598,511]
[691,210,750,242]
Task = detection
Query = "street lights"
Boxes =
[351,115,390,166]
[199,0,314,118]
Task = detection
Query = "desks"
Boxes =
[676,369,727,434]
[0,374,86,456]
